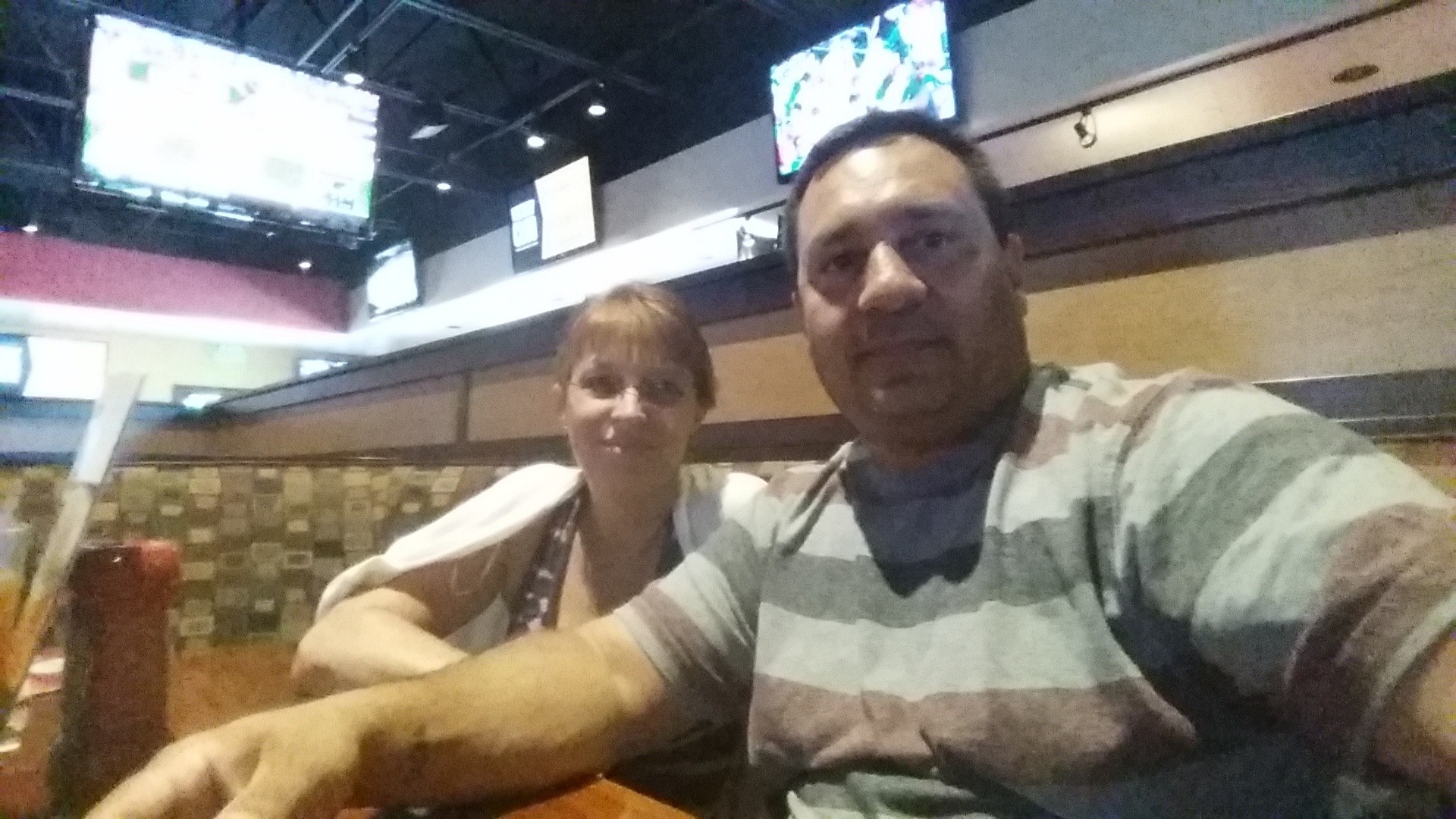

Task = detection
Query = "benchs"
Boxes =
[0,441,1456,643]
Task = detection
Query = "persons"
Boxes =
[291,281,787,819]
[86,110,1456,819]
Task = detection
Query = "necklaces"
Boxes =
[583,497,669,603]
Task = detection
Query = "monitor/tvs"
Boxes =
[768,0,962,183]
[365,238,420,318]
[76,10,381,235]
[505,153,600,272]
[0,333,111,401]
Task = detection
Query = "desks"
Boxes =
[0,644,694,818]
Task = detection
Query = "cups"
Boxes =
[0,516,33,669]
